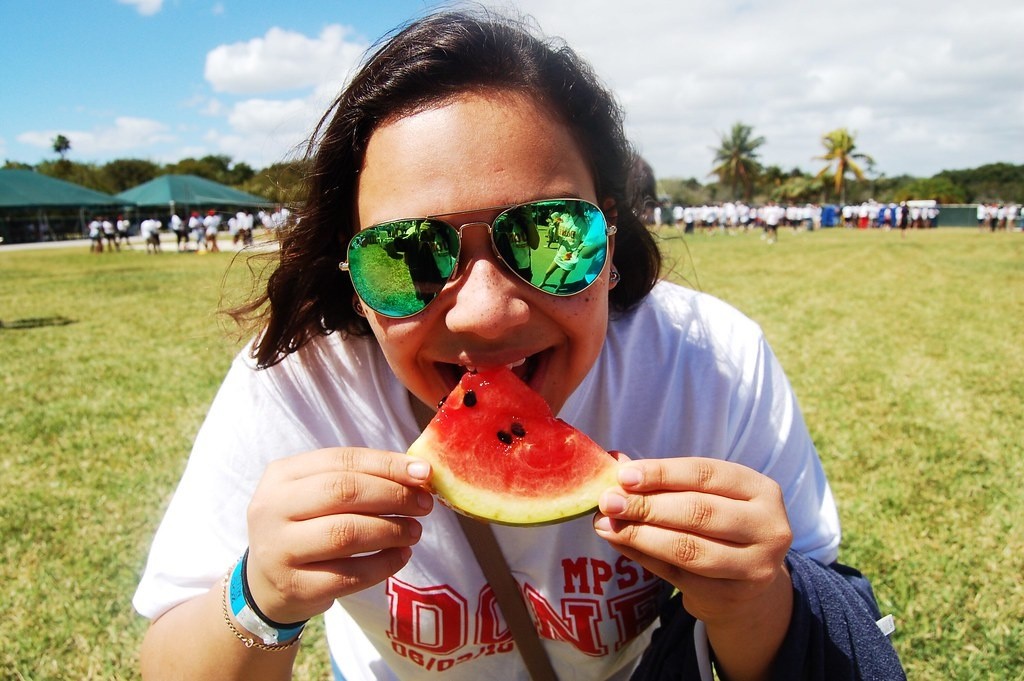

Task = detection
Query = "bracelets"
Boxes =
[222,546,309,651]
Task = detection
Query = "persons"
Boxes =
[89,203,290,252]
[132,12,840,681]
[977,201,1024,232]
[352,219,460,306]
[655,199,939,244]
[493,202,606,294]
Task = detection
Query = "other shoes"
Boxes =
[553,288,558,294]
[538,283,545,288]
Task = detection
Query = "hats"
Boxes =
[551,212,559,220]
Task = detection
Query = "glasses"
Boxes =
[338,197,617,319]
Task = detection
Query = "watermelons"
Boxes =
[405,366,622,528]
